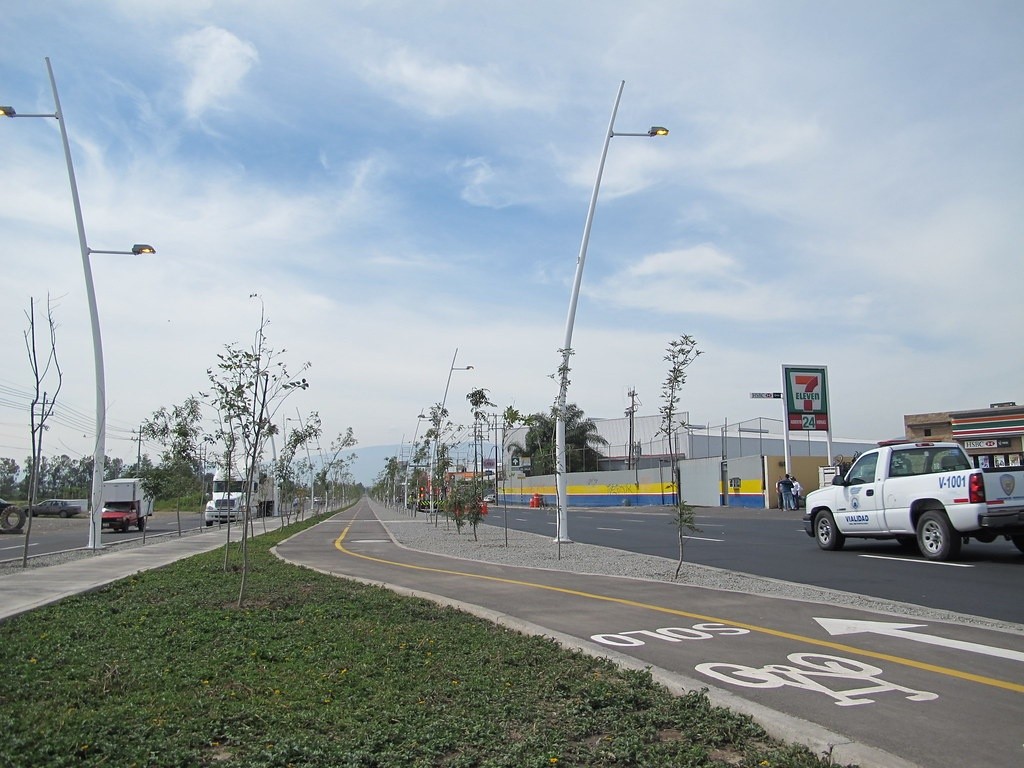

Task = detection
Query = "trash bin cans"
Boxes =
[529,493,544,507]
[259,500,274,516]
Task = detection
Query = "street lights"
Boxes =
[0,58,159,554]
[546,79,672,544]
[285,408,315,510]
[429,349,474,515]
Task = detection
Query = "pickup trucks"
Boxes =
[802,437,1024,562]
[21,498,82,520]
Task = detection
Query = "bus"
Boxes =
[476,474,497,504]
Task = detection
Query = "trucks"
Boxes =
[406,471,446,512]
[101,477,156,533]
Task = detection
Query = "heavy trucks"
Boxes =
[205,458,278,527]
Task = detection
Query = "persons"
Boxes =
[790,476,802,510]
[781,474,795,511]
[775,476,784,509]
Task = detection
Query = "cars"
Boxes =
[396,495,405,503]
[293,497,325,507]
[0,498,17,517]
